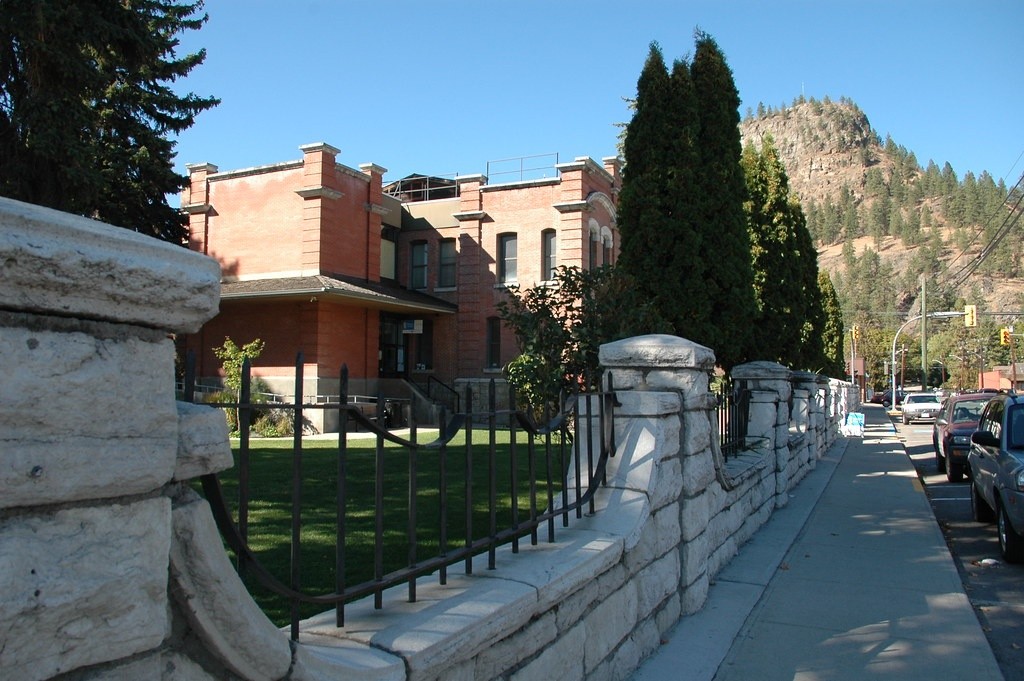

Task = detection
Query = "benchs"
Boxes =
[348,405,388,432]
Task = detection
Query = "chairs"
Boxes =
[954,407,976,422]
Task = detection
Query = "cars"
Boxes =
[933,391,1004,481]
[900,392,947,426]
[873,390,912,410]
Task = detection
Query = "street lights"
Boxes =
[891,311,970,412]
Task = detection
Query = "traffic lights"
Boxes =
[853,325,861,339]
[1001,330,1011,345]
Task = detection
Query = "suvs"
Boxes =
[966,388,1024,566]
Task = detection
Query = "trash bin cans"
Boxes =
[384,398,412,427]
